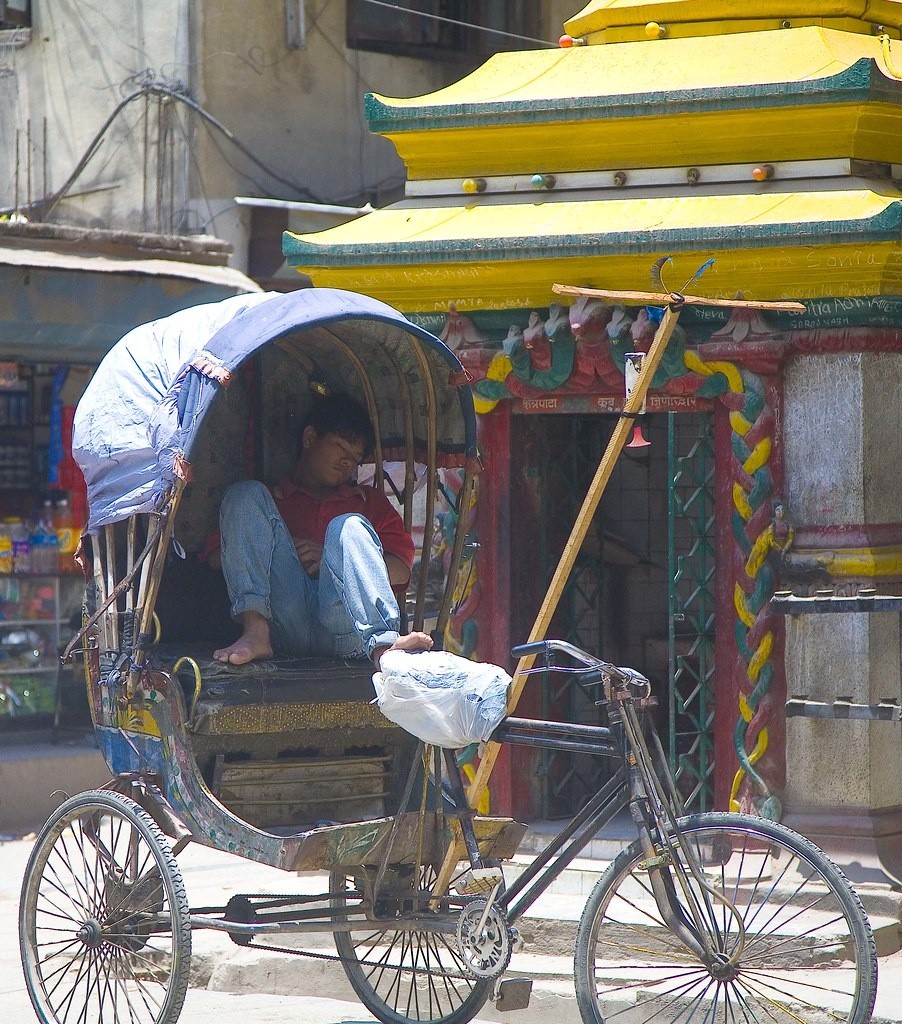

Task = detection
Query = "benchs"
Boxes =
[142,550,423,750]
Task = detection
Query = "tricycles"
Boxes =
[14,287,880,1024]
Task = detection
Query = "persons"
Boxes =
[197,395,434,673]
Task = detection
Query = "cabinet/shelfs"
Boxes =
[0,570,91,730]
[1,361,67,501]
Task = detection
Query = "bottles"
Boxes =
[1,498,74,573]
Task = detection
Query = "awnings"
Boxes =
[0,238,266,293]
